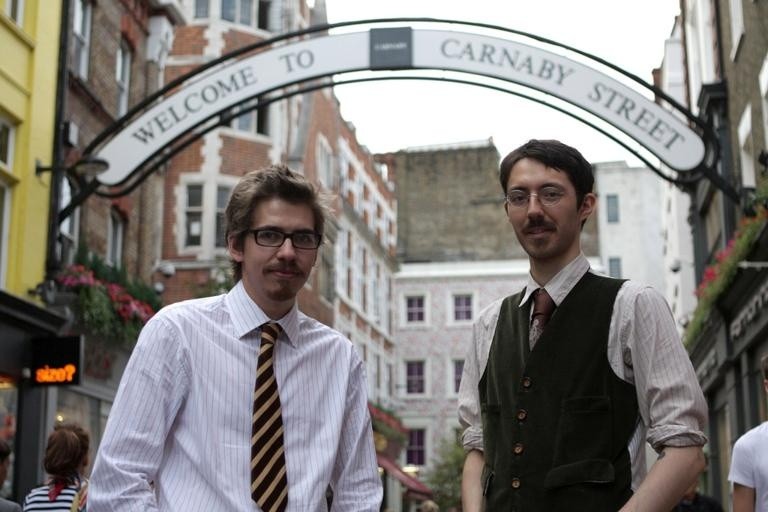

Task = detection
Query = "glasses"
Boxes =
[247,229,322,249]
[504,187,564,208]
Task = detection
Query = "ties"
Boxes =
[251,323,288,512]
[529,289,556,352]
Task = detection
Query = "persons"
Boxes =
[726,355,768,512]
[86,165,384,512]
[455,139,710,512]
[22,422,90,512]
[0,438,22,512]
